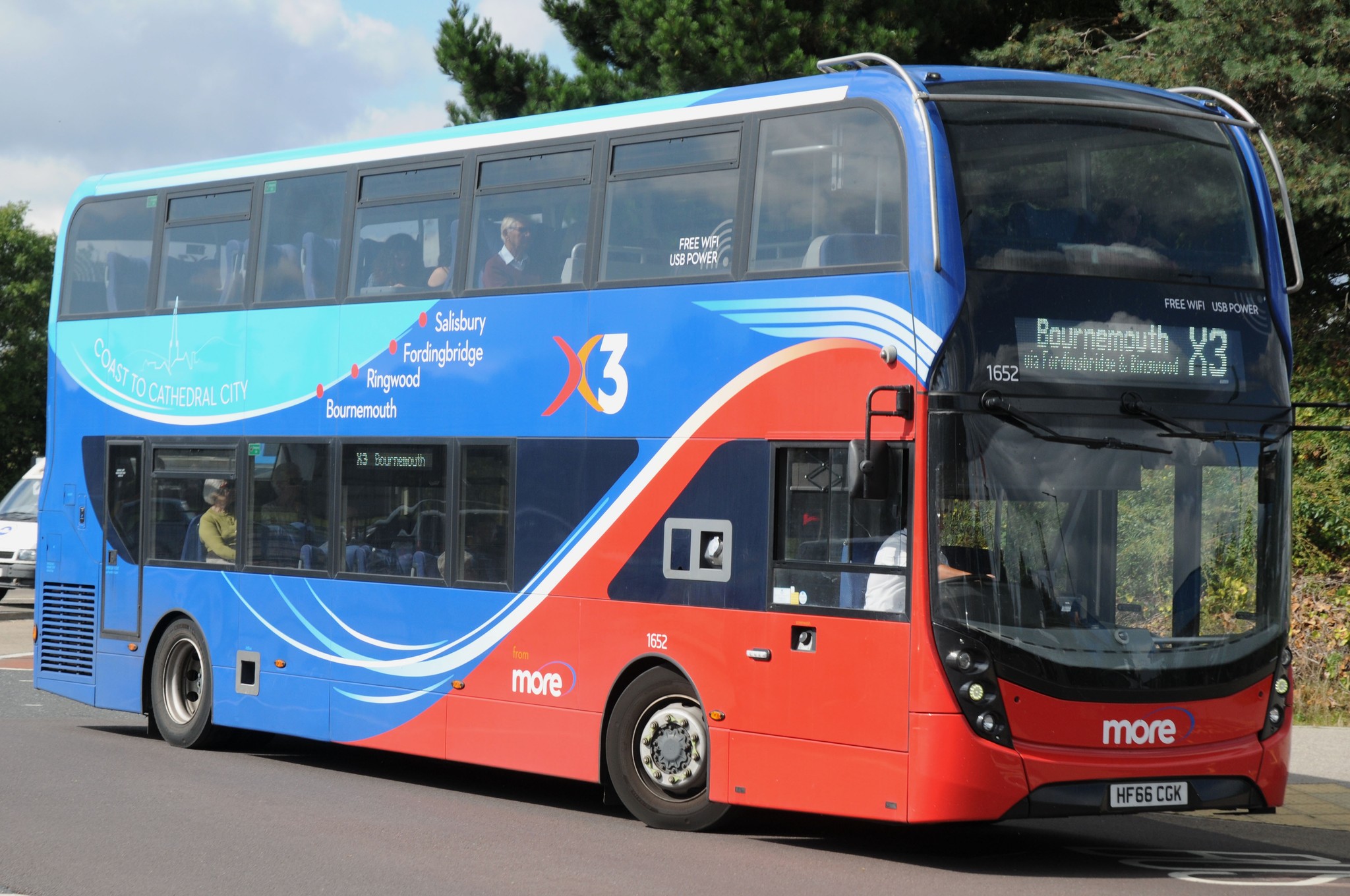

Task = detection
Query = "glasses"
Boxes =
[224,483,235,489]
[282,477,303,485]
[509,227,527,233]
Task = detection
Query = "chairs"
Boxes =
[840,542,882,610]
[179,514,506,583]
[106,230,902,310]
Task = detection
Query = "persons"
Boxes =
[483,211,540,287]
[367,233,453,291]
[437,550,477,581]
[261,464,312,527]
[199,478,237,565]
[863,498,996,613]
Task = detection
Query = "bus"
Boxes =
[31,53,1303,835]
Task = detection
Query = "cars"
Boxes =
[0,456,46,603]
